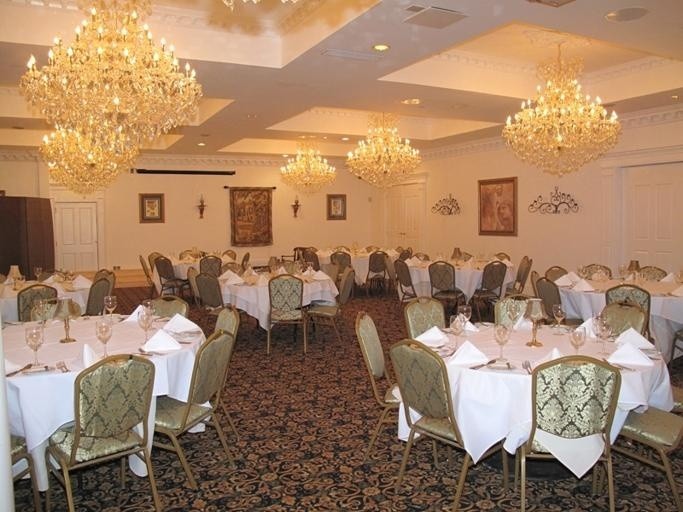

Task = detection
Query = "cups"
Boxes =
[619,265,627,278]
[592,311,606,329]
[674,270,681,283]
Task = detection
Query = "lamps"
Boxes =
[502,40,622,178]
[20,0,203,140]
[40,122,137,197]
[279,141,336,194]
[345,110,422,190]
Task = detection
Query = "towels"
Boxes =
[608,342,652,368]
[403,258,415,267]
[671,283,683,296]
[410,256,422,263]
[568,271,580,282]
[144,328,182,352]
[222,253,234,261]
[575,317,596,337]
[514,313,534,330]
[451,313,477,331]
[414,326,449,349]
[226,273,243,285]
[162,313,200,333]
[554,275,573,286]
[303,266,316,275]
[83,343,102,369]
[219,270,233,279]
[613,328,656,349]
[123,305,146,322]
[277,266,286,274]
[450,341,491,367]
[73,273,95,289]
[313,270,329,280]
[543,347,566,361]
[660,273,680,285]
[572,278,594,292]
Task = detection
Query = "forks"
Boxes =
[521,361,534,376]
[55,360,68,377]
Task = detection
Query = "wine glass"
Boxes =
[137,313,154,347]
[142,300,155,314]
[213,248,222,259]
[307,262,312,278]
[102,295,117,327]
[95,323,114,360]
[597,322,616,357]
[32,267,41,281]
[459,305,470,334]
[568,327,587,356]
[295,262,302,281]
[450,315,466,354]
[62,269,77,283]
[191,246,197,260]
[33,298,46,324]
[600,271,608,290]
[578,264,587,279]
[552,303,568,336]
[23,324,47,374]
[492,321,510,367]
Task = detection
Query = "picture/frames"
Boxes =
[326,193,346,221]
[225,186,277,247]
[478,176,518,237]
[138,193,165,224]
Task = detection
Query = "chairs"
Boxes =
[355,310,405,466]
[304,268,354,345]
[153,296,189,319]
[11,433,43,512]
[18,285,56,322]
[222,250,236,259]
[330,252,355,298]
[494,294,535,327]
[266,275,309,354]
[398,250,409,262]
[638,266,666,282]
[514,355,621,512]
[473,261,507,322]
[392,339,509,512]
[43,270,66,281]
[530,271,541,298]
[149,252,162,274]
[597,302,647,342]
[495,253,509,262]
[303,250,319,271]
[406,296,445,339]
[200,257,222,277]
[504,255,533,300]
[196,273,249,341]
[582,265,611,282]
[179,250,195,260]
[139,256,155,301]
[385,256,399,298]
[428,261,465,322]
[241,252,250,272]
[93,270,116,295]
[214,306,238,439]
[81,279,110,318]
[545,266,566,282]
[273,260,299,275]
[31,299,79,320]
[394,260,420,307]
[155,256,193,302]
[152,330,234,486]
[597,405,683,512]
[537,278,583,327]
[45,355,163,512]
[365,251,389,296]
[606,285,654,343]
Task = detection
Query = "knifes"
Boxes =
[468,360,497,372]
[5,364,34,378]
